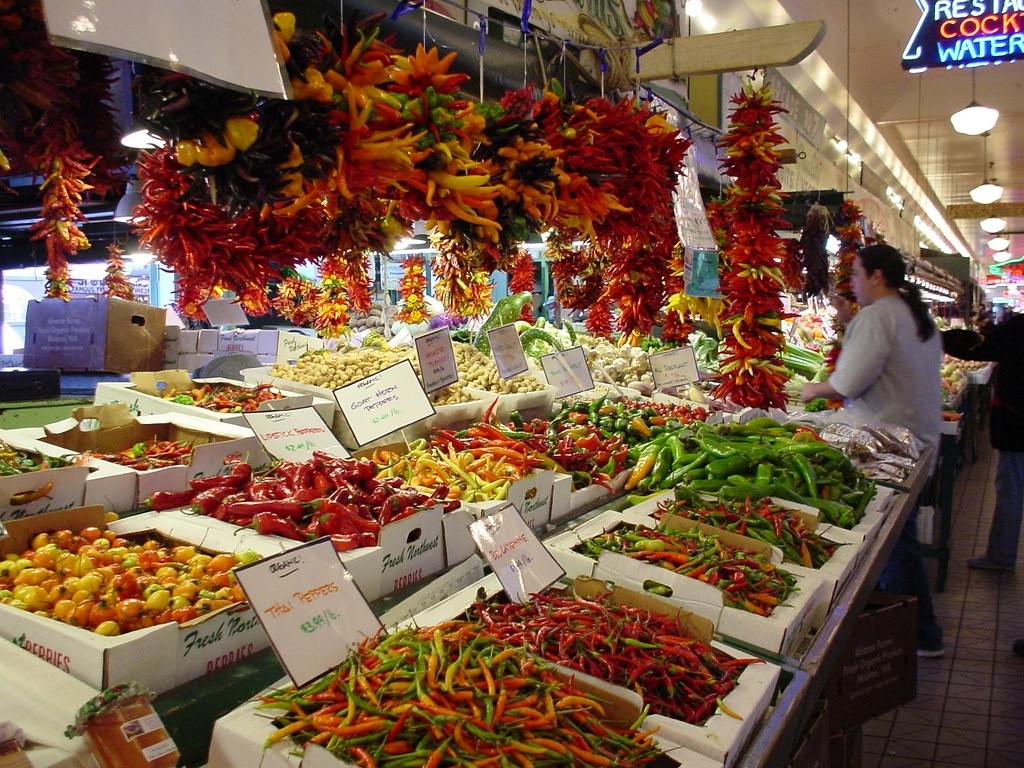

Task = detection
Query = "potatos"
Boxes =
[953,361,982,371]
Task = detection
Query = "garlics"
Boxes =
[551,329,651,382]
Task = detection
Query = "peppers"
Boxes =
[0,381,877,768]
[0,0,725,346]
[713,77,869,411]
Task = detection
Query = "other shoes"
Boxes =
[1013,639,1024,657]
[966,554,1016,572]
[918,625,947,657]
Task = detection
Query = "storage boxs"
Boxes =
[2,295,997,768]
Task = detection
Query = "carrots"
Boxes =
[944,411,963,421]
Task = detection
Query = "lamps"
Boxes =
[980,205,1011,263]
[949,67,998,137]
[968,132,1005,204]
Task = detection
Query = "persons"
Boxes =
[975,304,991,331]
[941,312,1024,570]
[803,244,946,658]
[998,306,1013,323]
